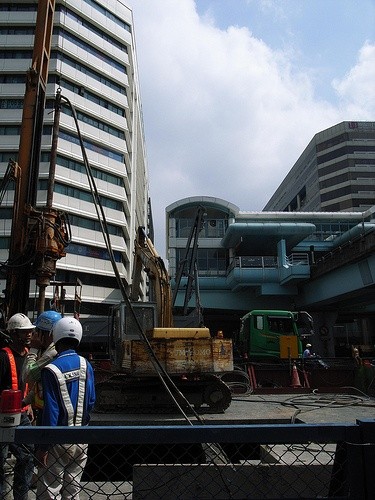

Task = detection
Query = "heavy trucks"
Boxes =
[235,309,375,393]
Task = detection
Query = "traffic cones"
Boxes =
[288,363,303,388]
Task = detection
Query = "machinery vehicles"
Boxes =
[88,224,235,416]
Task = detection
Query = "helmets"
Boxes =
[54,317,82,344]
[6,313,36,330]
[36,311,62,331]
[306,344,312,348]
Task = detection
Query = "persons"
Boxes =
[0,313,35,500]
[21,311,62,490]
[303,344,312,369]
[34,317,95,500]
[351,344,363,369]
